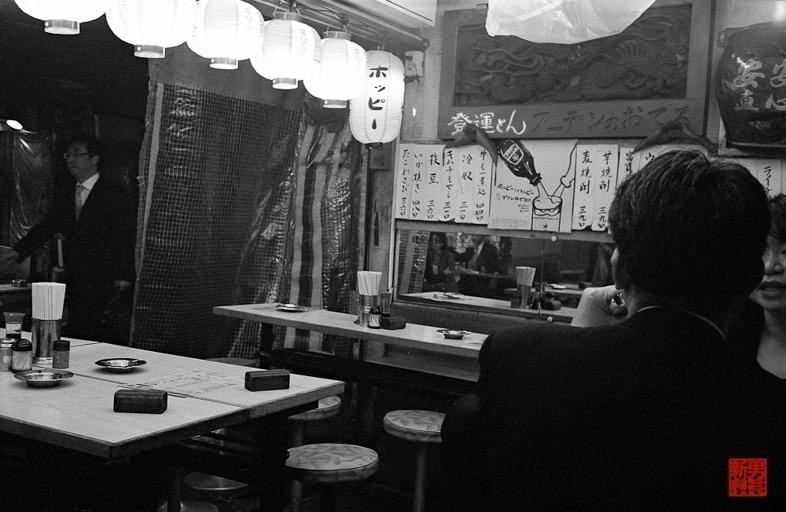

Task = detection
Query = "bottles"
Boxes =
[496,140,542,185]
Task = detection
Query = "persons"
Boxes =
[457,232,498,288]
[2,136,133,343]
[439,148,786,511]
[747,191,786,386]
[424,232,454,290]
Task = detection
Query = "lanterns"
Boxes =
[485,2,655,44]
[187,1,263,70]
[255,12,321,89]
[305,30,367,108]
[106,0,201,58]
[12,0,105,36]
[349,48,405,149]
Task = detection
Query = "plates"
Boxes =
[13,369,73,388]
[436,329,472,340]
[93,358,147,374]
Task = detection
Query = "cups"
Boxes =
[29,316,65,358]
[353,293,379,325]
[516,284,532,309]
[3,312,25,340]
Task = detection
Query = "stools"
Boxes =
[290,396,344,511]
[384,410,443,512]
[285,443,379,512]
[180,465,259,512]
[207,357,257,368]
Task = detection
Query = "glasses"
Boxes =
[63,150,93,163]
